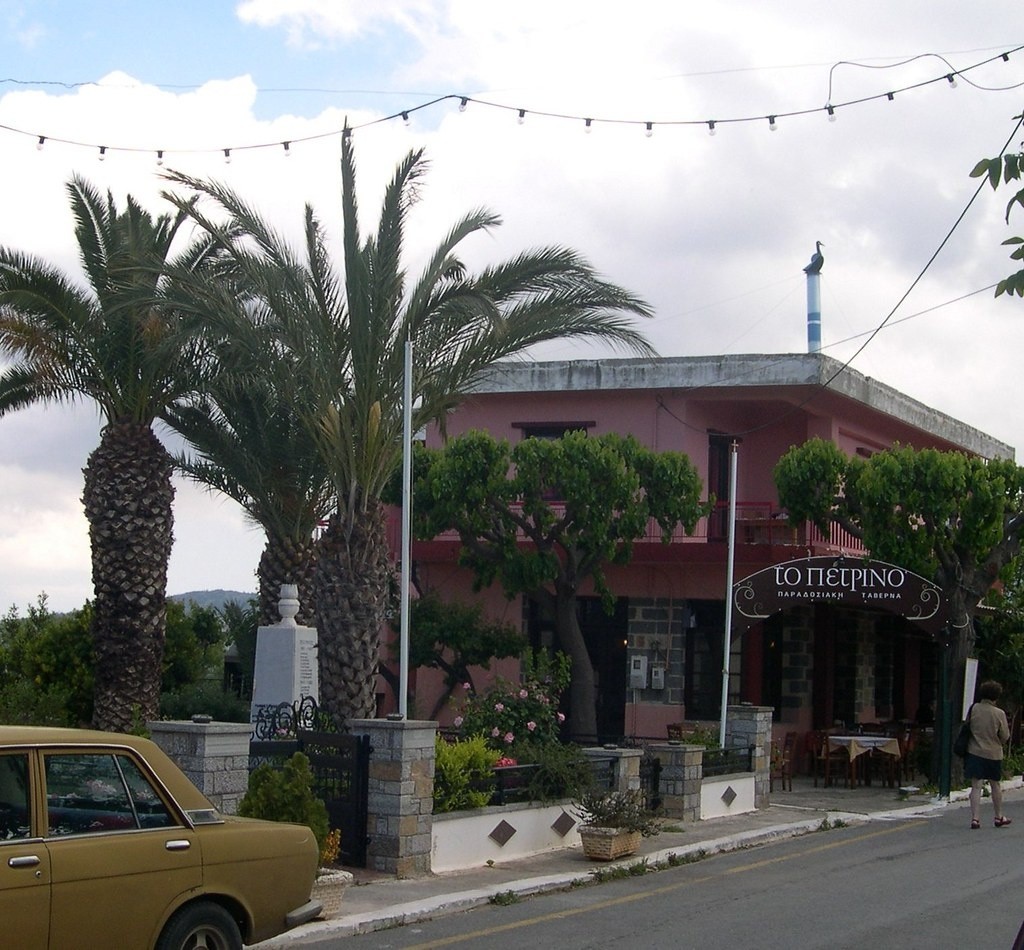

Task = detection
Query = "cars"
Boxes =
[0,726,323,950]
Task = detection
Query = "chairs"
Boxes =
[774,732,799,792]
[811,731,857,789]
[844,720,923,786]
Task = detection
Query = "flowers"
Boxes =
[447,645,572,767]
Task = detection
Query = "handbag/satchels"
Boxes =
[954,702,975,758]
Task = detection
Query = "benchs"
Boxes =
[10,803,169,832]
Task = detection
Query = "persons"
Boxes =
[962,680,1013,829]
[915,699,937,726]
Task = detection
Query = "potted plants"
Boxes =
[570,784,662,861]
[237,751,355,921]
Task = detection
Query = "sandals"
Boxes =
[995,816,1012,826]
[971,819,980,828]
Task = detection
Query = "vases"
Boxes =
[471,772,524,806]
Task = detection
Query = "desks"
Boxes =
[821,736,901,788]
